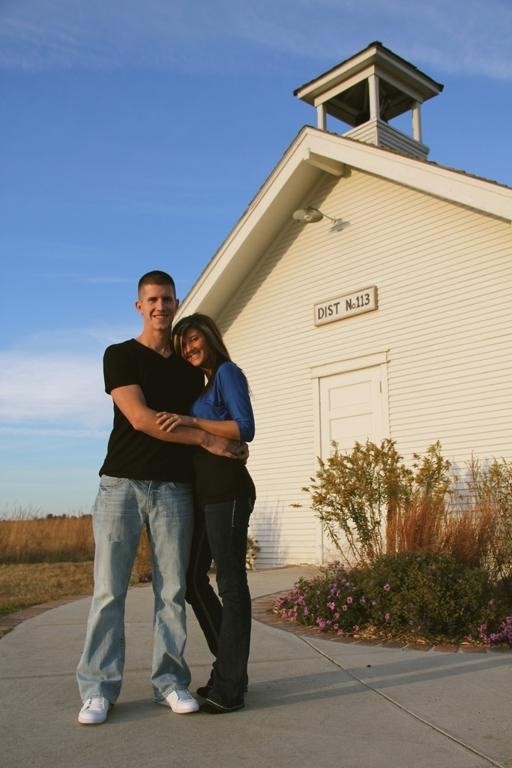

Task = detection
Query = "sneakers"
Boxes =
[78,696,113,724]
[156,685,248,714]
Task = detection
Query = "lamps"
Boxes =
[293,205,338,224]
